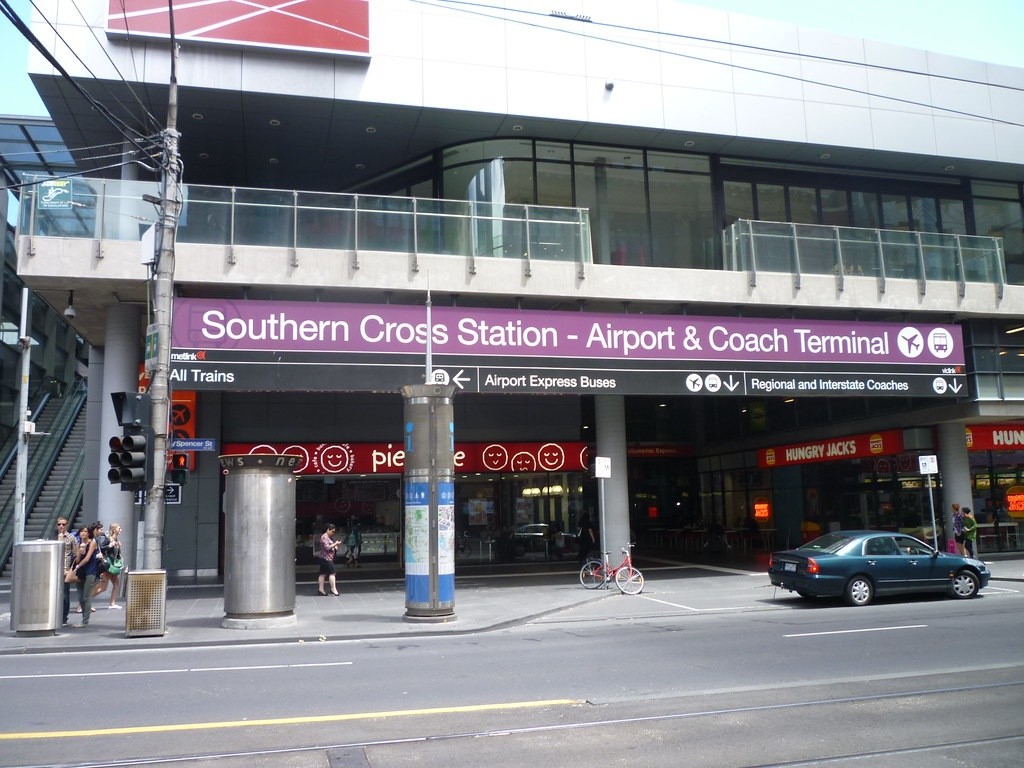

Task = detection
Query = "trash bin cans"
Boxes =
[9,539,65,637]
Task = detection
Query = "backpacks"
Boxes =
[348,528,356,546]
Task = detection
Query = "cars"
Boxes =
[767,529,993,607]
[511,524,565,556]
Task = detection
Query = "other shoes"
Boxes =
[75,623,88,627]
[356,565,361,568]
[108,604,122,609]
[62,621,68,626]
[76,607,83,613]
[90,607,96,612]
[347,561,350,568]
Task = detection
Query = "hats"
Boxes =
[962,507,970,514]
[952,503,960,510]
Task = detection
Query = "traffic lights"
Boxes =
[119,435,149,485]
[110,391,153,426]
[170,452,190,485]
[107,434,127,486]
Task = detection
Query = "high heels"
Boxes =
[318,590,328,596]
[330,590,340,596]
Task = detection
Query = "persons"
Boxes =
[72,521,124,627]
[950,503,977,558]
[576,512,595,562]
[343,518,362,568]
[996,501,1010,548]
[317,523,341,596]
[56,517,81,626]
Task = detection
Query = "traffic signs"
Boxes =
[162,481,183,504]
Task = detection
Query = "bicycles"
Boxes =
[579,542,645,596]
[456,529,473,558]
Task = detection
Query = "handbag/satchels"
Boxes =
[65,559,80,584]
[107,557,123,576]
[96,558,110,575]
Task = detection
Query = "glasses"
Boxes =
[331,530,336,533]
[57,523,66,526]
